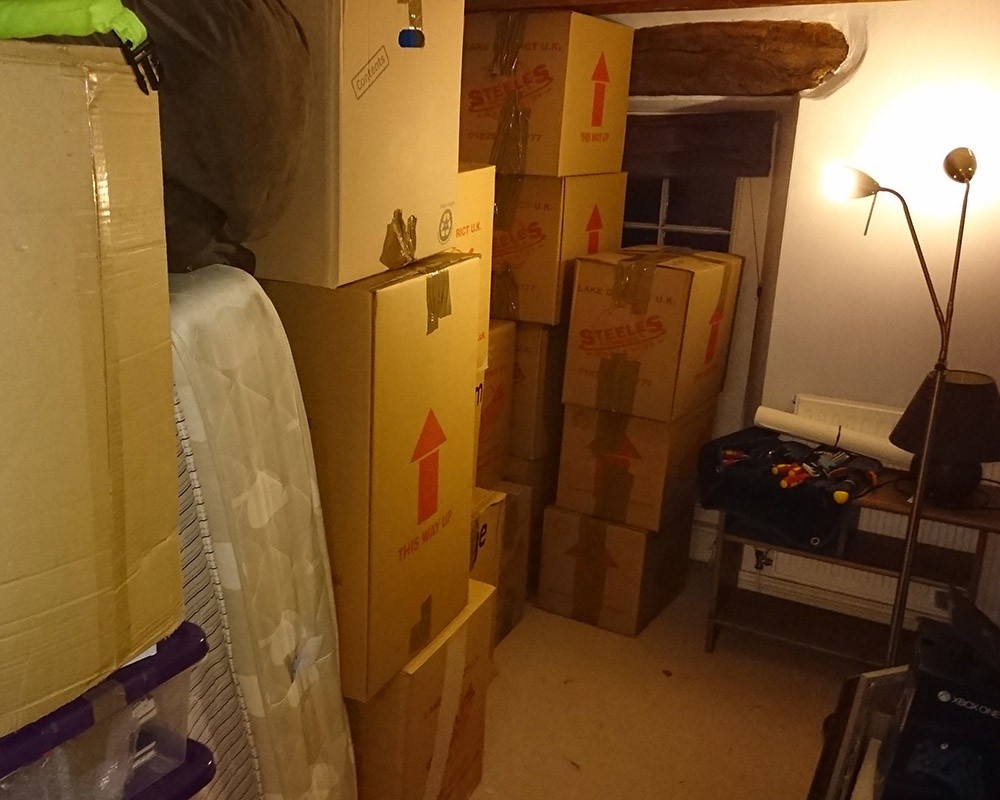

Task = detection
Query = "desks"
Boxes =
[704,467,1000,674]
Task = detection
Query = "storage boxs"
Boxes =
[0,0,749,799]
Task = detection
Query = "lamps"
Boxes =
[889,369,999,496]
[817,144,977,673]
